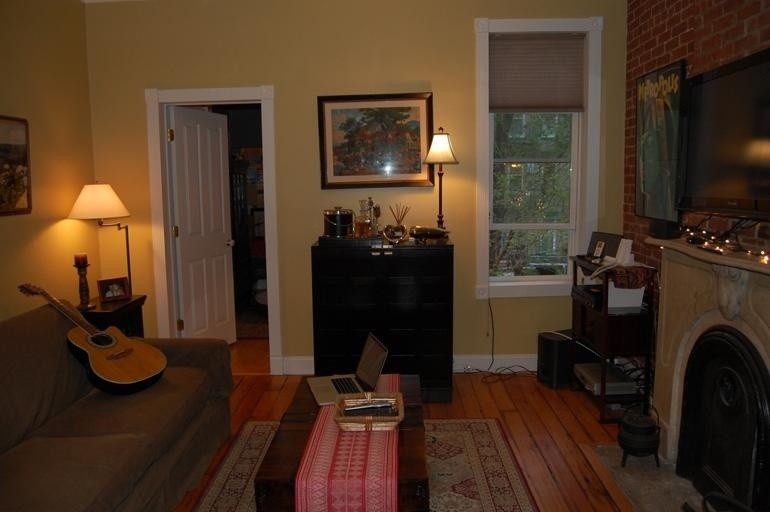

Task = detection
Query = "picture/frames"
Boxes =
[0,113,35,219]
[96,274,132,305]
[627,54,693,229]
[315,88,436,192]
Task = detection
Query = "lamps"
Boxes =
[420,125,462,232]
[65,177,140,293]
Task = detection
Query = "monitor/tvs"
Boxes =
[673,46,770,222]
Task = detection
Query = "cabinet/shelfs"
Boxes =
[75,292,149,339]
[565,253,660,425]
[309,236,457,403]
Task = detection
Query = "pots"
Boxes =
[321,206,356,238]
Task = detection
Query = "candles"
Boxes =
[72,253,87,266]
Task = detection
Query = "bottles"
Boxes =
[354,200,378,240]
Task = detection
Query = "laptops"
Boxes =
[306,332,389,407]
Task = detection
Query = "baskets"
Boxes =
[332,391,405,433]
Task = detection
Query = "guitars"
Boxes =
[18,282,168,394]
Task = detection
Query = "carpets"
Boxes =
[189,410,541,511]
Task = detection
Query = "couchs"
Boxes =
[0,299,235,511]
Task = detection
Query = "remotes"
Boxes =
[344,400,390,412]
